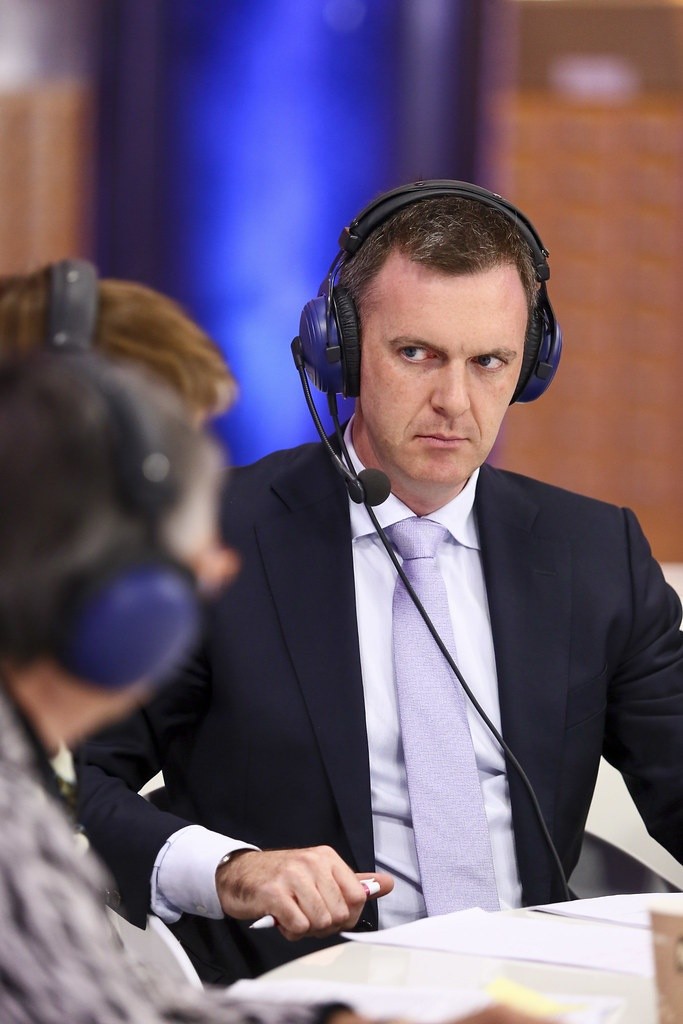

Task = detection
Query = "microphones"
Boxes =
[295,356,391,506]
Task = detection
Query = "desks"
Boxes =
[254,893,683,1024]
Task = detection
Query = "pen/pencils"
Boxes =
[249,877,381,930]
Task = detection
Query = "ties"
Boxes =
[380,519,500,918]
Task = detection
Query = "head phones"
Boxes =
[57,354,198,697]
[289,180,560,408]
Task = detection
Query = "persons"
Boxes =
[63,176,683,982]
[0,256,563,1024]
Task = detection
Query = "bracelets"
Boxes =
[221,854,231,864]
